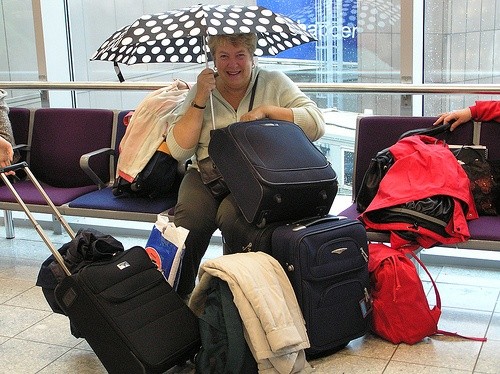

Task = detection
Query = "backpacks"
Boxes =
[366,242,441,346]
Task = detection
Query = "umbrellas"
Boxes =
[90,3,318,130]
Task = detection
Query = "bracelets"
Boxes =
[192,102,206,109]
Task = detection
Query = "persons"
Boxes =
[0,89,21,189]
[165,33,326,295]
[433,100,500,131]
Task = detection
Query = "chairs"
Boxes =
[336,115,500,262]
[0,107,187,240]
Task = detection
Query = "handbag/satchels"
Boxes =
[144,212,191,292]
[452,147,498,215]
[357,123,452,214]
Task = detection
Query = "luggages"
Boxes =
[1,160,201,374]
[207,117,338,228]
[271,213,372,357]
[111,136,179,200]
